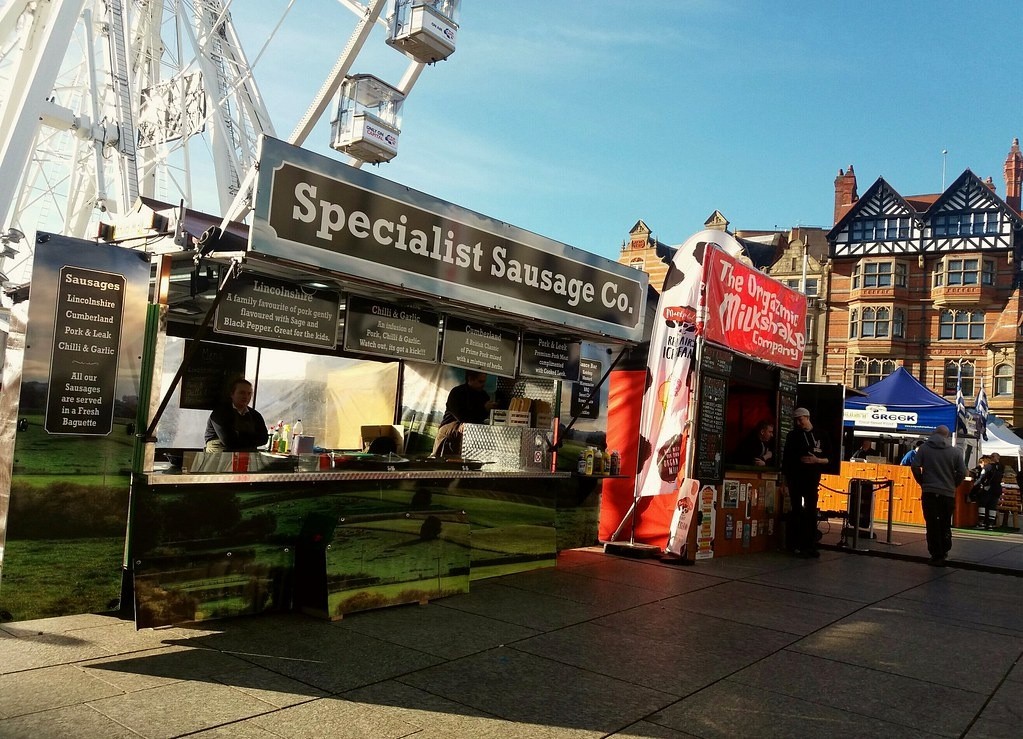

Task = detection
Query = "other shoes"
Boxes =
[988,525,994,530]
[927,557,946,566]
[973,524,986,529]
[804,548,820,558]
[788,544,810,558]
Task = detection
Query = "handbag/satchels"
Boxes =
[969,478,984,501]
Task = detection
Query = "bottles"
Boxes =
[265,425,279,452]
[578,445,621,477]
[291,419,303,451]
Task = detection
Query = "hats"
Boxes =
[791,407,810,417]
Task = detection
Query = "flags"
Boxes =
[974,373,989,419]
[956,362,966,418]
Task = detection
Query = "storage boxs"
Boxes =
[360,425,405,454]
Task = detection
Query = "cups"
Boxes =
[233,452,250,473]
[277,441,286,452]
[319,453,330,471]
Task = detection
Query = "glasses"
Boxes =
[475,377,486,384]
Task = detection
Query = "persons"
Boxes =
[781,407,832,559]
[1016,458,1023,493]
[911,425,967,567]
[965,453,1002,530]
[852,438,877,459]
[732,421,774,467]
[430,370,497,460]
[205,380,269,453]
[900,441,925,466]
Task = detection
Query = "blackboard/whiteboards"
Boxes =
[212,265,341,351]
[343,295,440,364]
[180,339,247,410]
[778,367,799,393]
[440,314,520,380]
[700,342,736,377]
[778,391,797,471]
[848,480,872,529]
[518,329,582,383]
[694,371,729,485]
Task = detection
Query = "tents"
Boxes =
[952,422,1023,471]
[842,366,957,433]
